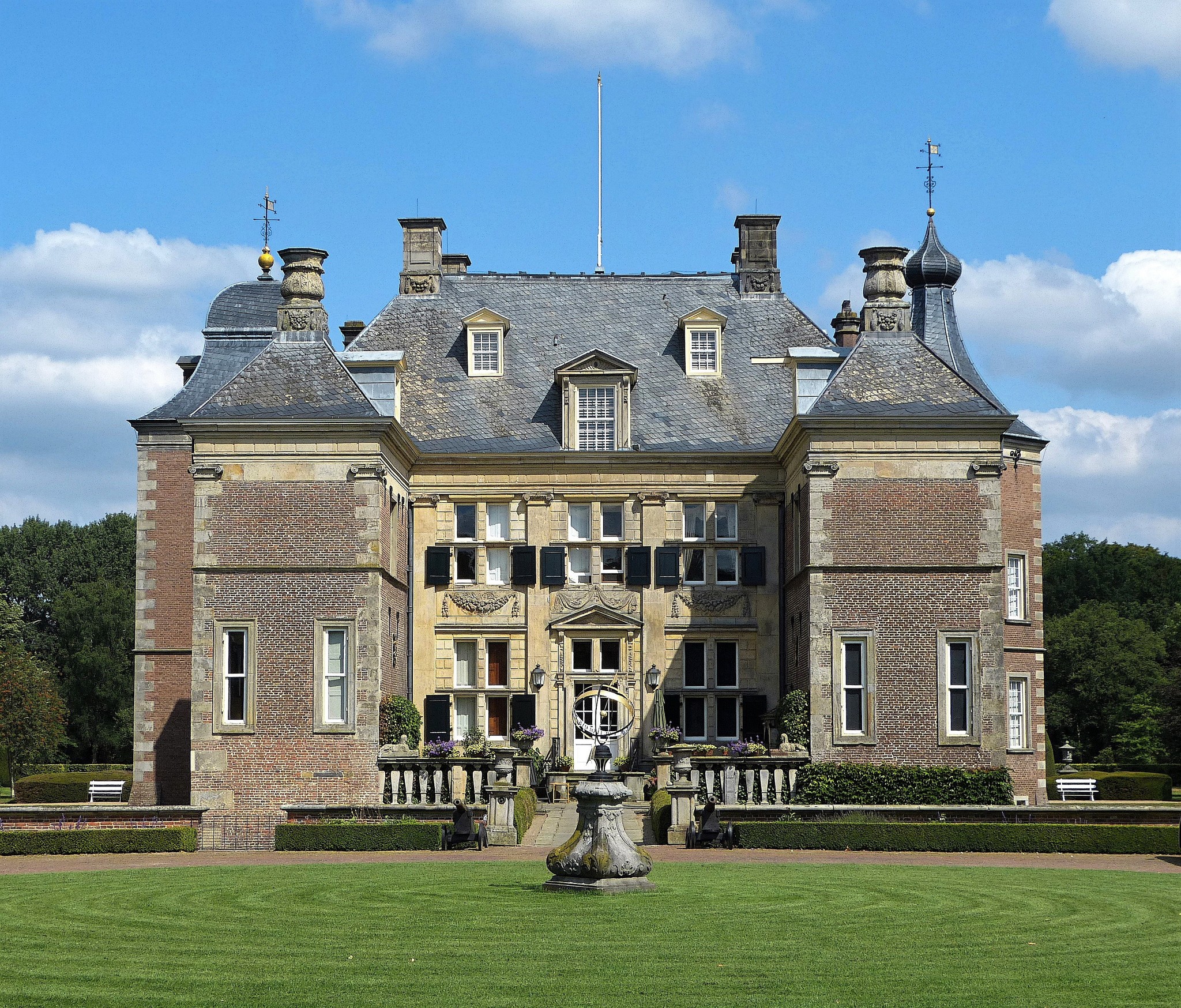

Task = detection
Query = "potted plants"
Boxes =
[691,743,717,757]
[718,746,729,756]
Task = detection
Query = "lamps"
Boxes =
[646,662,661,693]
[530,661,546,694]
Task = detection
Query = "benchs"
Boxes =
[1055,778,1099,801]
[88,780,126,802]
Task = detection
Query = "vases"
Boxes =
[560,766,571,772]
[619,764,630,772]
[518,740,534,749]
[644,792,652,801]
[751,750,763,756]
[653,738,670,746]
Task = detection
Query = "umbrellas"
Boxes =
[652,688,667,753]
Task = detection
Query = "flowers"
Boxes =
[645,768,658,788]
[464,735,497,761]
[727,735,768,755]
[648,721,682,742]
[510,722,545,741]
[612,756,629,767]
[421,736,461,758]
[555,755,575,767]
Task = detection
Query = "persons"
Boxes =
[401,734,408,745]
[781,733,788,743]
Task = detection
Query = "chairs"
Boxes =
[530,763,550,804]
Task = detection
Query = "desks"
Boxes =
[545,771,568,803]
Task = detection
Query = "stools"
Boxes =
[549,783,570,804]
